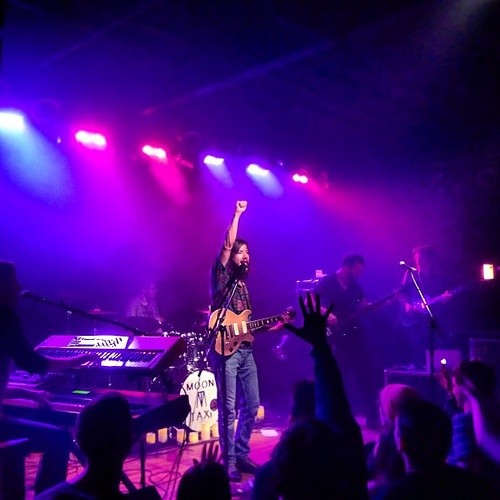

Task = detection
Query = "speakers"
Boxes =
[423,347,462,373]
[384,369,445,408]
[468,337,500,366]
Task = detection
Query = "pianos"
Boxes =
[2,330,191,492]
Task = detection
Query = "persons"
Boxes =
[210,199,290,483]
[0,246,500,500]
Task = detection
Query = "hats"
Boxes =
[379,383,418,421]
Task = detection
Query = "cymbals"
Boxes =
[80,308,124,316]
[116,317,161,331]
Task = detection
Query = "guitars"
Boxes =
[324,275,422,346]
[392,278,480,330]
[209,305,299,358]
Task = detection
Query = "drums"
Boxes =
[148,364,219,433]
[160,330,208,385]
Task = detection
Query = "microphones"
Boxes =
[400,261,418,271]
[241,258,249,268]
[20,291,38,300]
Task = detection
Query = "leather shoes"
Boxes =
[229,471,242,482]
[236,457,259,473]
